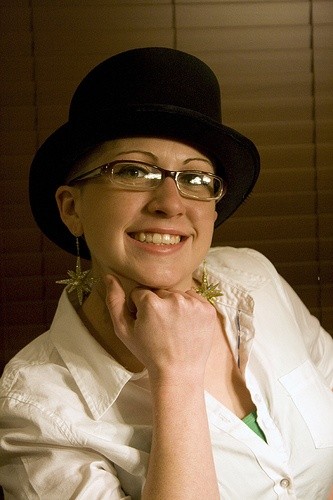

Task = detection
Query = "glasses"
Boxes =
[65,159,226,207]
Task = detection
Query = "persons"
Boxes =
[1,45,333,500]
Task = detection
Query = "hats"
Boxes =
[27,46,262,262]
